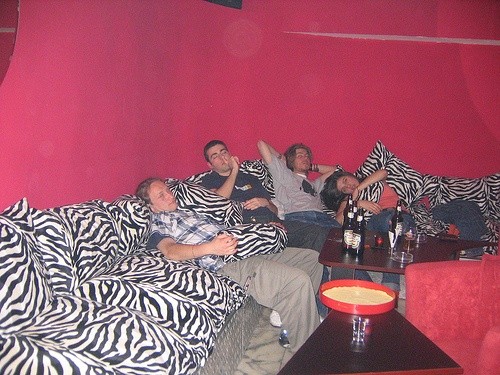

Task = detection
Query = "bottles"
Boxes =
[342,192,366,259]
[391,201,405,242]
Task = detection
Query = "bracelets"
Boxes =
[192,245,196,258]
[277,153,282,160]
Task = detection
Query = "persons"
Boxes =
[136,178,325,355]
[201,141,329,349]
[255,138,374,328]
[321,170,489,308]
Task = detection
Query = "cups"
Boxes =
[352,316,366,342]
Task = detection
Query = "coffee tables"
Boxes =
[275,309,465,375]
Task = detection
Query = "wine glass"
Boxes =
[402,227,417,253]
[387,220,402,260]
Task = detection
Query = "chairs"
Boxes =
[404,252,500,375]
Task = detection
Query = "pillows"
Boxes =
[419,175,488,237]
[484,171,500,231]
[28,206,82,299]
[57,199,122,282]
[239,159,276,199]
[353,138,425,207]
[113,193,153,255]
[0,194,57,338]
[165,176,244,229]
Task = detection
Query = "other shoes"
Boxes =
[269,310,282,326]
[320,315,326,323]
[279,329,290,348]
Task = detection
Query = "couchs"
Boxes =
[0,171,500,375]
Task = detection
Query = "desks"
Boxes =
[317,226,463,281]
